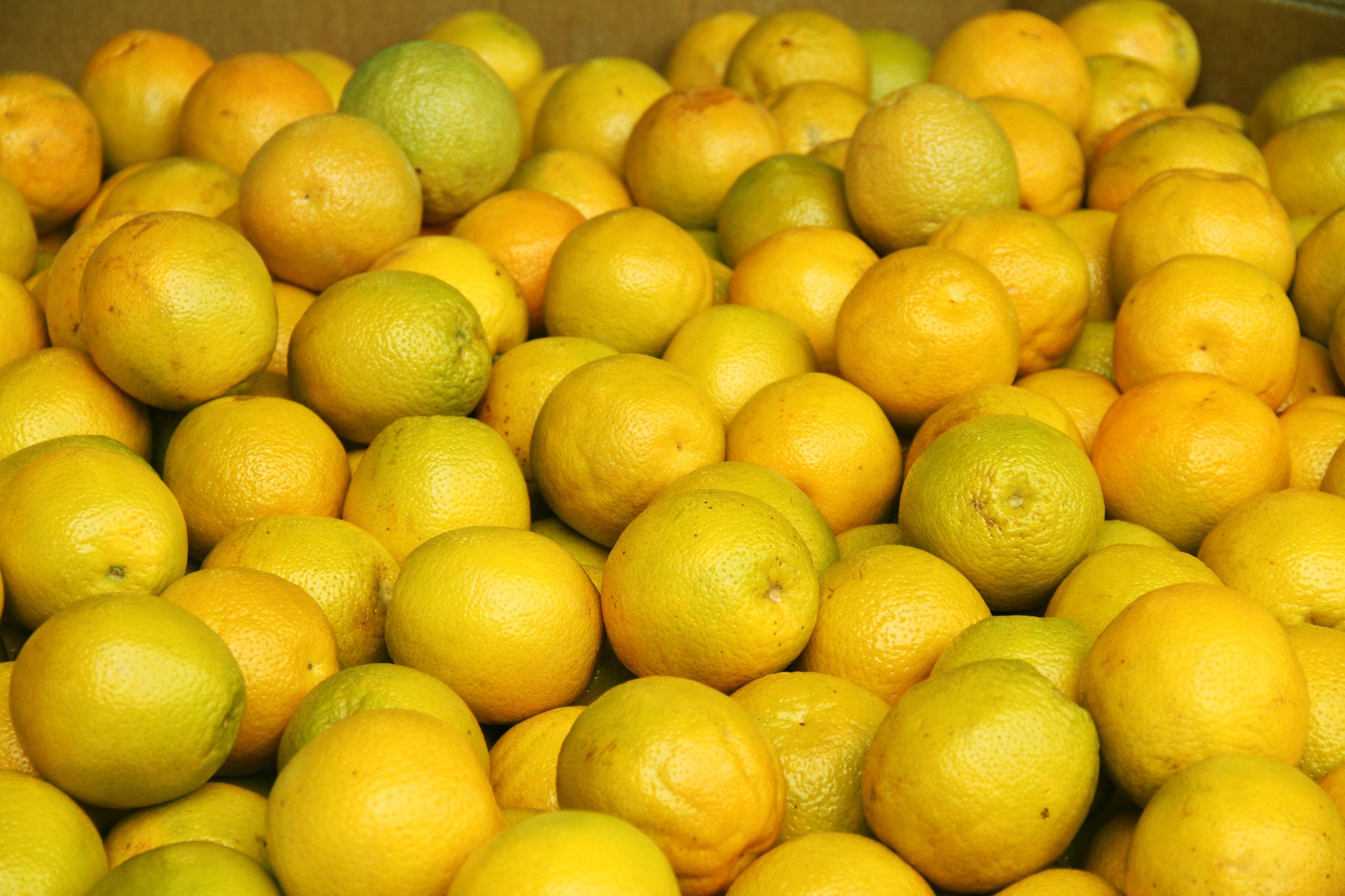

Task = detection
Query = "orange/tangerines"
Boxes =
[0,0,1342,896]
